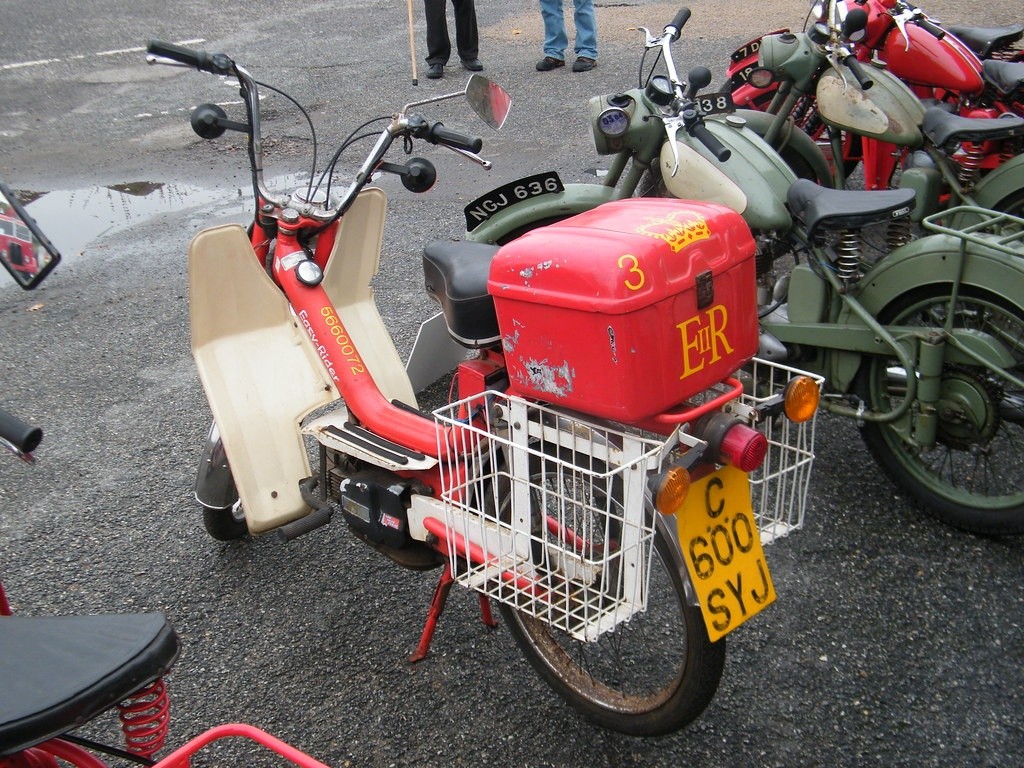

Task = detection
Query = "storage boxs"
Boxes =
[485,196,759,426]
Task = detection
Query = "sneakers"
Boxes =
[572,56,595,72]
[536,55,565,71]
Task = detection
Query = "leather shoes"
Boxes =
[427,63,443,78]
[462,56,483,71]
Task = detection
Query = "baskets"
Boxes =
[432,390,662,643]
[690,356,825,545]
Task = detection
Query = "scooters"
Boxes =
[147,40,828,739]
[0,185,331,768]
[456,1,1024,540]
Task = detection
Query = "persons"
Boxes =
[536,0,598,72]
[424,0,483,79]
